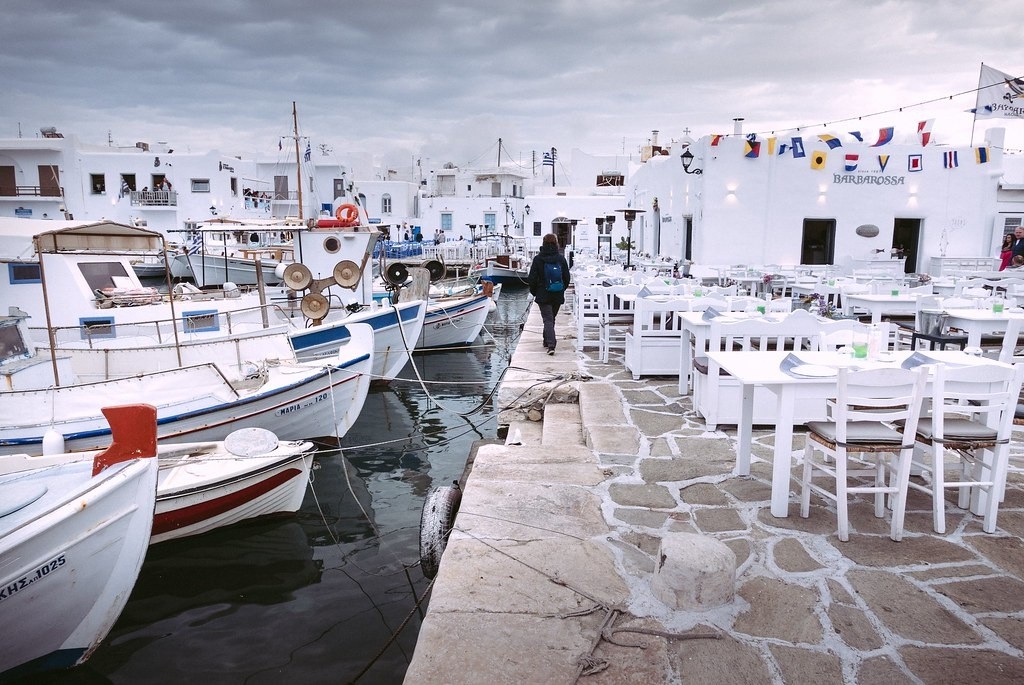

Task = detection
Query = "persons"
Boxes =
[379,229,464,245]
[244,186,270,211]
[1000,226,1024,270]
[139,177,172,203]
[529,233,571,355]
[249,232,293,263]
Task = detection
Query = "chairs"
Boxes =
[579,254,1024,542]
[373,237,498,260]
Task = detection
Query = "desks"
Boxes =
[574,256,1024,517]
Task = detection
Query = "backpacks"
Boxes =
[543,253,564,292]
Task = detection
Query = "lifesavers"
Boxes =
[336,204,358,223]
[418,484,462,580]
[347,207,368,226]
[102,287,159,297]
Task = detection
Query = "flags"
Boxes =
[868,127,894,147]
[767,137,806,158]
[817,134,843,150]
[744,140,761,158]
[878,155,890,173]
[943,151,959,168]
[916,118,936,147]
[908,154,923,172]
[974,65,1024,120]
[543,152,553,165]
[849,132,863,142]
[506,204,515,219]
[845,155,859,171]
[278,140,282,151]
[711,135,720,147]
[304,140,312,162]
[811,151,827,169]
[975,147,990,165]
[121,179,129,193]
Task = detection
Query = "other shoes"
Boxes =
[544,342,557,355]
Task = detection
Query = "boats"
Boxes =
[0,210,502,454]
[150,428,319,547]
[466,222,532,288]
[0,402,157,685]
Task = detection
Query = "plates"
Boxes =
[709,316,739,322]
[789,365,838,377]
[922,363,949,375]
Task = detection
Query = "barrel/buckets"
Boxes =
[918,310,949,336]
